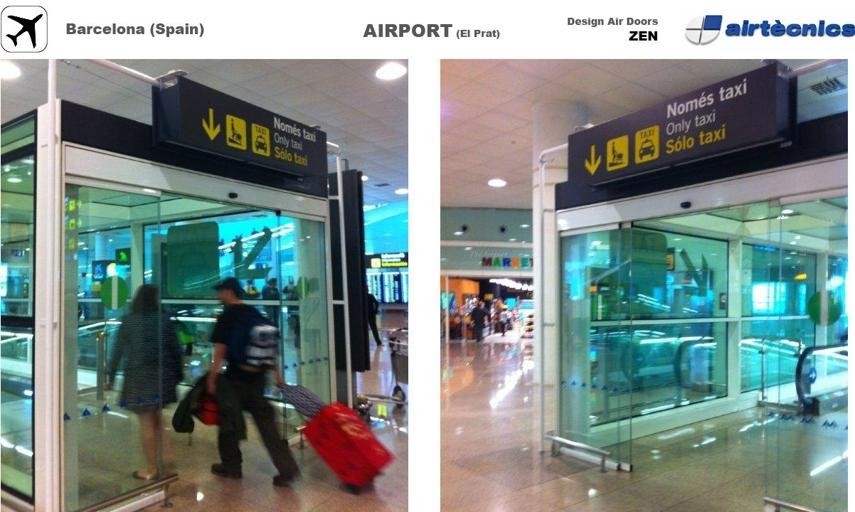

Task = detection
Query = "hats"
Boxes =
[212,277,246,295]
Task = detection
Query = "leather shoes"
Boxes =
[211,463,242,477]
[273,464,301,486]
[133,467,158,480]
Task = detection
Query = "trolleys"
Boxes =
[386,326,408,408]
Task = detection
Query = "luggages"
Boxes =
[274,381,392,492]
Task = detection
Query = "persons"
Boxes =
[366,283,384,348]
[206,277,304,487]
[103,282,184,480]
[291,278,326,377]
[497,307,510,337]
[468,301,493,344]
[261,279,282,328]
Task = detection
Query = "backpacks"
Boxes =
[236,318,280,370]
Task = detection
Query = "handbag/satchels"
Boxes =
[278,381,324,418]
[195,398,220,424]
